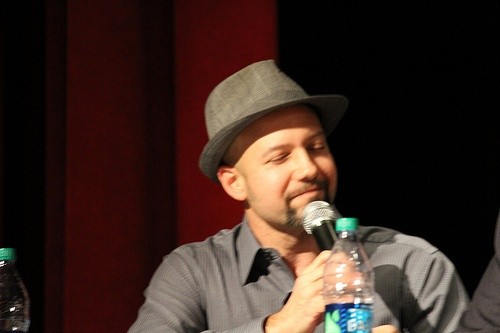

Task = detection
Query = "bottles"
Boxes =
[0,245,31,333]
[324,217,377,333]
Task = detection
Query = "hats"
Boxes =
[199,59,348,181]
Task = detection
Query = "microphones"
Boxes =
[305,200,340,252]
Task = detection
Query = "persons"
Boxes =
[126,59,469,333]
[461,212,500,333]
